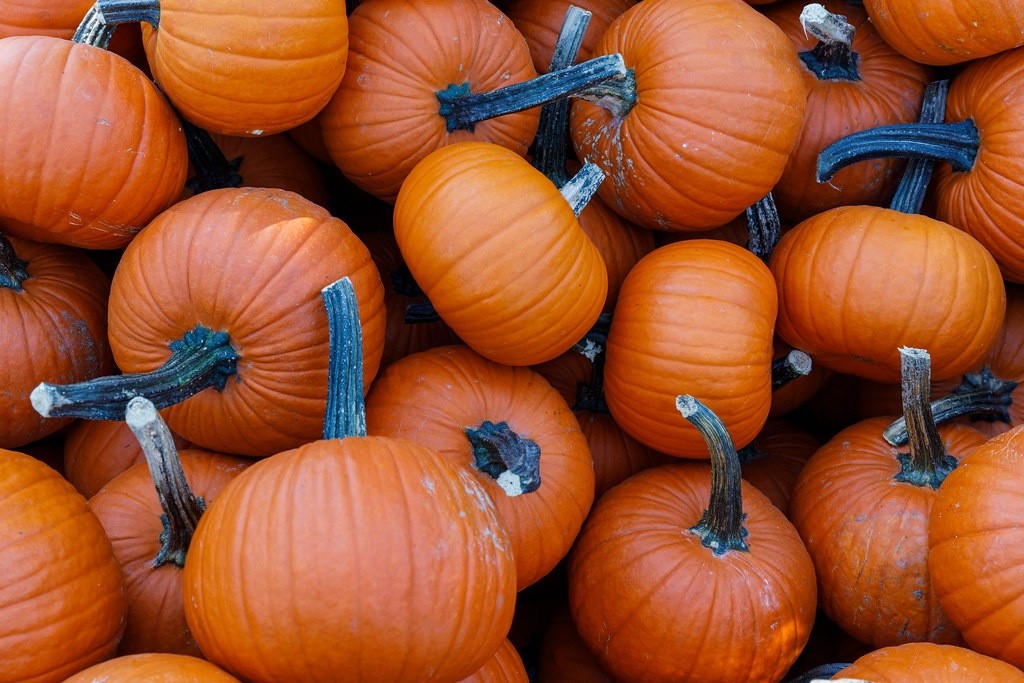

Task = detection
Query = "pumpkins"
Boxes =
[0,0,1024,683]
